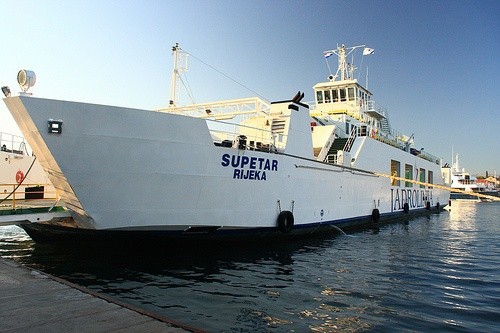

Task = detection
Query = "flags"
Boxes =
[363,47,374,55]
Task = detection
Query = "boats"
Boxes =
[1,132,71,222]
[441,153,499,200]
[1,42,450,252]
